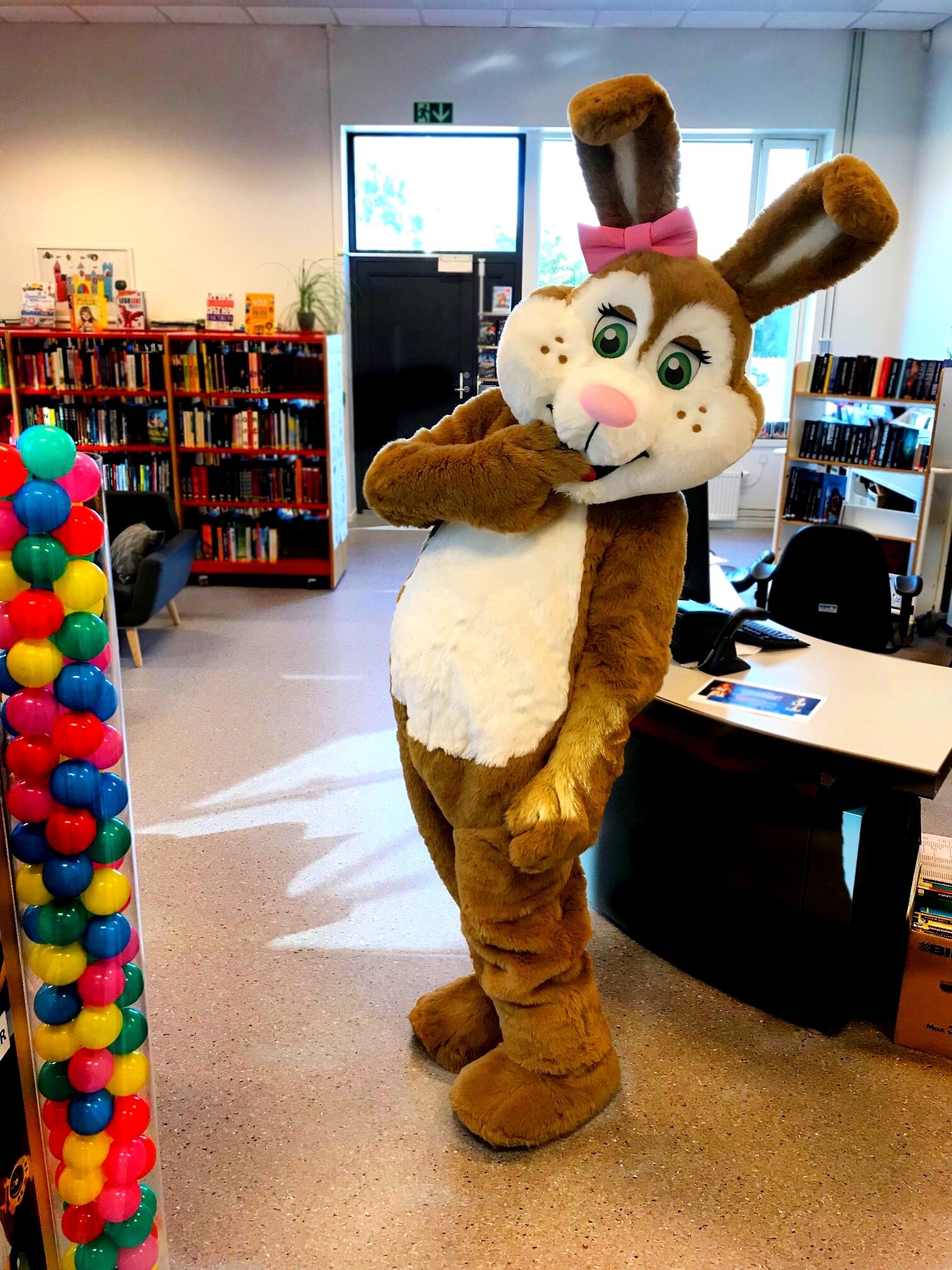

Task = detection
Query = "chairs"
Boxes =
[83,490,199,667]
[725,522,924,655]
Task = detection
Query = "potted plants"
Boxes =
[263,256,349,335]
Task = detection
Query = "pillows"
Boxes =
[110,523,165,583]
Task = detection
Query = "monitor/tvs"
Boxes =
[678,483,709,603]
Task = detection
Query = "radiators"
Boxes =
[708,470,751,521]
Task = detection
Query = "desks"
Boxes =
[580,550,952,1041]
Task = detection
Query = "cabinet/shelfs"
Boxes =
[0,324,347,590]
[772,354,952,638]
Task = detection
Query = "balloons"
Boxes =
[0,424,160,1270]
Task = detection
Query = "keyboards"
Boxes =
[705,603,811,647]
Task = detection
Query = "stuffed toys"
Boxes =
[360,75,899,1152]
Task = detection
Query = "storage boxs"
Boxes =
[894,862,952,1059]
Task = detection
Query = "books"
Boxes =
[20,280,55,328]
[205,289,235,332]
[779,353,952,526]
[115,291,148,330]
[71,293,108,334]
[0,336,328,564]
[245,292,275,337]
